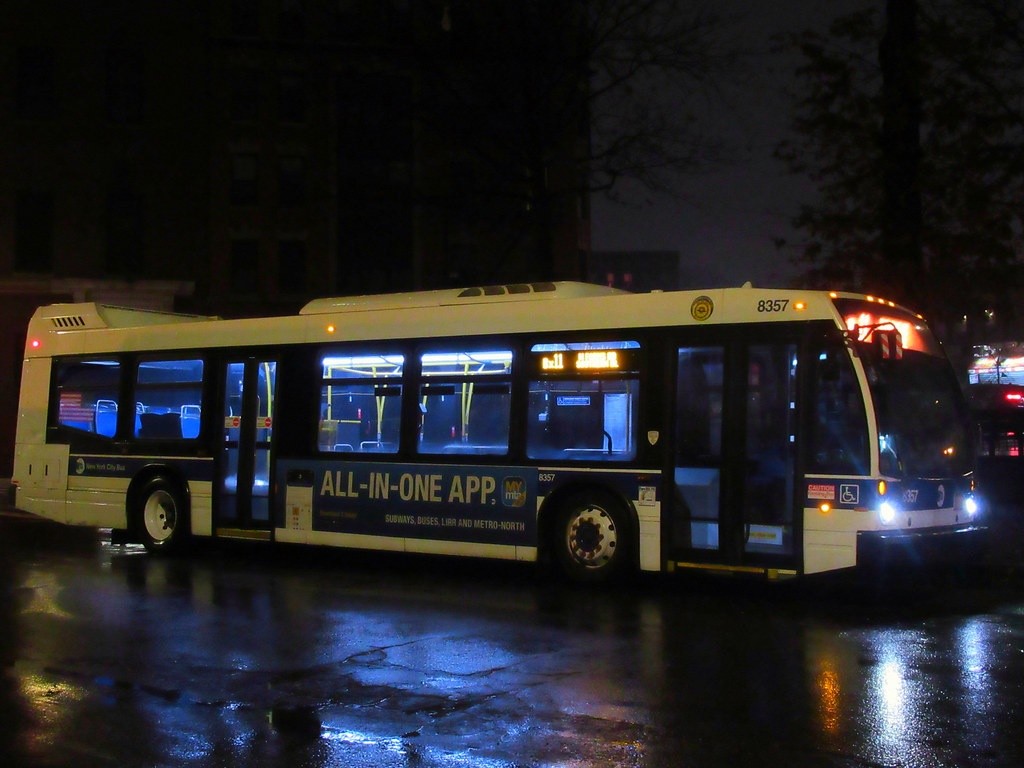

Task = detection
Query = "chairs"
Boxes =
[59,399,202,438]
[333,441,385,452]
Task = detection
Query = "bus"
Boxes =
[10,280,991,596]
